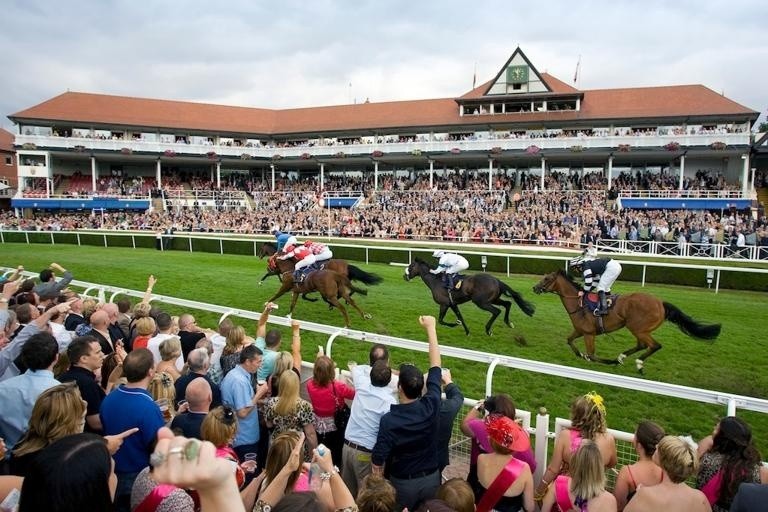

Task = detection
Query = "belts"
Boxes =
[345,440,373,453]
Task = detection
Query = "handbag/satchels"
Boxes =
[334,403,350,430]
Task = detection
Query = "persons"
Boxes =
[464,107,487,115]
[25,129,147,142]
[0,171,768,282]
[0,263,768,512]
[161,137,213,146]
[221,125,743,148]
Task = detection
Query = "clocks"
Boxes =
[506,65,529,84]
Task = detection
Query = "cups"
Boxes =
[257,379,265,399]
[154,397,171,421]
[244,453,258,472]
[178,399,188,412]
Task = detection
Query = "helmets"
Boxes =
[305,241,312,247]
[569,256,585,269]
[432,251,444,258]
[287,246,295,252]
[275,231,282,237]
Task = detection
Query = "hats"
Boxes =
[485,413,530,452]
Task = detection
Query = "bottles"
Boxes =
[307,448,326,491]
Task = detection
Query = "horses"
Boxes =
[403,256,536,336]
[532,268,721,375]
[259,242,383,310]
[264,252,367,329]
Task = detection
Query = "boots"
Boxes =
[293,270,301,283]
[444,274,454,291]
[596,290,610,315]
